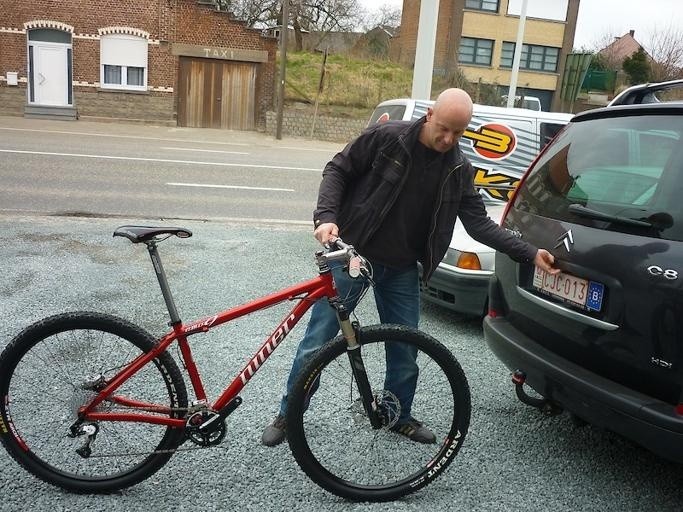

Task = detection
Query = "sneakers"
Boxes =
[262,414,288,448]
[381,413,436,444]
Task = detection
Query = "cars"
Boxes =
[500,95,542,112]
[483,75,683,462]
[361,97,576,322]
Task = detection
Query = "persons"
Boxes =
[258,86,563,449]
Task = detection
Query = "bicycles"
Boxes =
[0,225,471,499]
